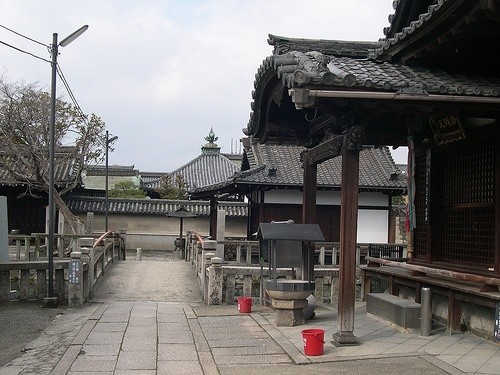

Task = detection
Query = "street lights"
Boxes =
[44,23,88,308]
[105,130,117,232]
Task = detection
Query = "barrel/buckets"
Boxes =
[301,328,326,356]
[237,297,253,313]
